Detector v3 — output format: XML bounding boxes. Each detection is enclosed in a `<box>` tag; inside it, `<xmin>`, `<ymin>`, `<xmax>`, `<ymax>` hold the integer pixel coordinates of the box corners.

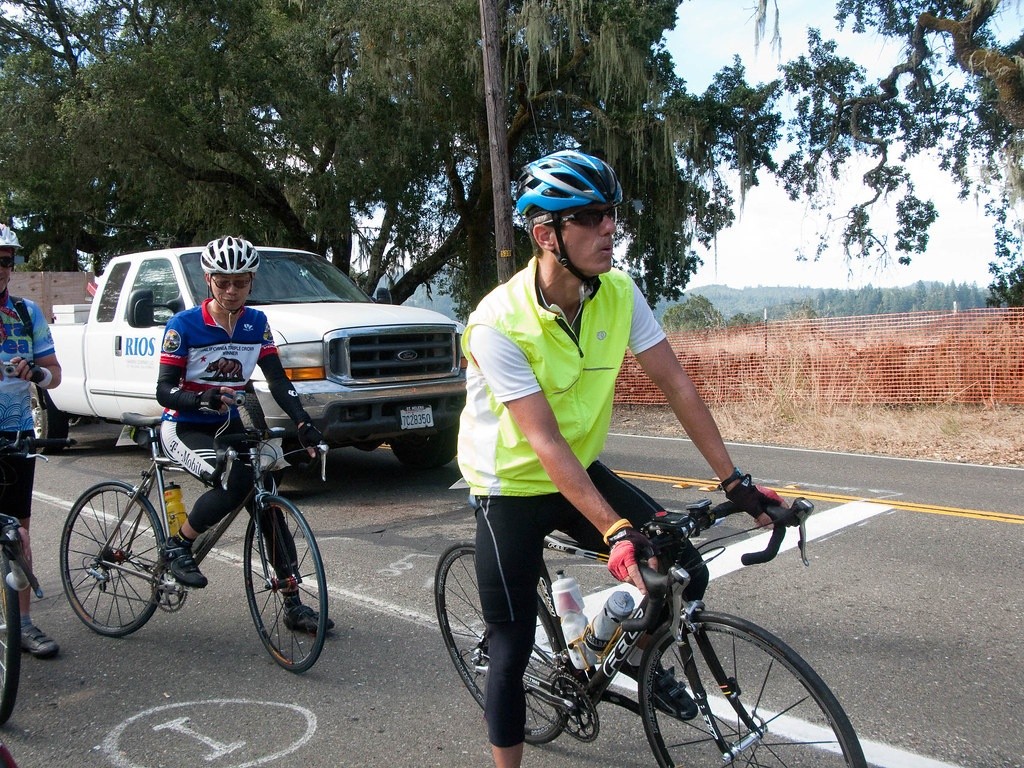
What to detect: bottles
<box><xmin>588</xmin><ymin>592</ymin><xmax>634</xmax><ymax>653</ymax></box>
<box><xmin>163</xmin><ymin>481</ymin><xmax>186</xmax><ymax>537</ymax></box>
<box><xmin>551</xmin><ymin>568</ymin><xmax>598</xmax><ymax>668</ymax></box>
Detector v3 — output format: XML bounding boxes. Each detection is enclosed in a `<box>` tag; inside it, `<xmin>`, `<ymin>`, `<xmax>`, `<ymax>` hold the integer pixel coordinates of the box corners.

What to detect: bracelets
<box><xmin>604</xmin><ymin>516</ymin><xmax>633</xmax><ymax>545</ymax></box>
<box><xmin>33</xmin><ymin>367</ymin><xmax>53</xmax><ymax>388</ymax></box>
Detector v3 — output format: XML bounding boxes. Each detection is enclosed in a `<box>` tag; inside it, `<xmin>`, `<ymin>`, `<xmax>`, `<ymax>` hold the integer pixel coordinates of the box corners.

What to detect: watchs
<box><xmin>717</xmin><ymin>466</ymin><xmax>746</xmax><ymax>492</ymax></box>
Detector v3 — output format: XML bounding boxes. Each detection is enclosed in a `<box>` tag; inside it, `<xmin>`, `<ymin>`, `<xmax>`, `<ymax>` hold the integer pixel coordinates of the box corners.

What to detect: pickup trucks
<box><xmin>46</xmin><ymin>246</ymin><xmax>467</xmax><ymax>490</ymax></box>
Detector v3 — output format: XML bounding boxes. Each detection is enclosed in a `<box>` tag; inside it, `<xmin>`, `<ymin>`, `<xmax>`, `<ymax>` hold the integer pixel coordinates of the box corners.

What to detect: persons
<box><xmin>156</xmin><ymin>235</ymin><xmax>336</xmax><ymax>632</ymax></box>
<box><xmin>0</xmin><ymin>218</ymin><xmax>62</xmax><ymax>659</ymax></box>
<box><xmin>457</xmin><ymin>148</ymin><xmax>795</xmax><ymax>768</ymax></box>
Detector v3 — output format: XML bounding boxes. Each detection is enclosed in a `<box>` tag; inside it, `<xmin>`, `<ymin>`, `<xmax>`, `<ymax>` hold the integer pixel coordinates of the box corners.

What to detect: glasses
<box><xmin>540</xmin><ymin>206</ymin><xmax>615</xmax><ymax>226</ymax></box>
<box><xmin>211</xmin><ymin>276</ymin><xmax>253</xmax><ymax>289</ymax></box>
<box><xmin>0</xmin><ymin>256</ymin><xmax>15</xmax><ymax>267</ymax></box>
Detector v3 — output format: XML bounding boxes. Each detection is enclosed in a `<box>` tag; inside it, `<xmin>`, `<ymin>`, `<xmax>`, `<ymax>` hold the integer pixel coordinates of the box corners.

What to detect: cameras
<box><xmin>0</xmin><ymin>362</ymin><xmax>19</xmax><ymax>378</ymax></box>
<box><xmin>224</xmin><ymin>390</ymin><xmax>247</xmax><ymax>406</ymax></box>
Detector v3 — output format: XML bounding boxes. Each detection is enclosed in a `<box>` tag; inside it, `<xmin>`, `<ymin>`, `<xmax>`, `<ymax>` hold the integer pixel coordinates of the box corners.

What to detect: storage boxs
<box><xmin>52</xmin><ymin>304</ymin><xmax>92</xmax><ymax>323</ymax></box>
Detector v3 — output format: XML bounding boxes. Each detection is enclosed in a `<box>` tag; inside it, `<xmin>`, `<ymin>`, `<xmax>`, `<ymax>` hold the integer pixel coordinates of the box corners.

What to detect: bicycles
<box><xmin>0</xmin><ymin>428</ymin><xmax>75</xmax><ymax>727</ymax></box>
<box><xmin>434</xmin><ymin>496</ymin><xmax>869</xmax><ymax>768</ymax></box>
<box><xmin>59</xmin><ymin>412</ymin><xmax>329</xmax><ymax>674</ymax></box>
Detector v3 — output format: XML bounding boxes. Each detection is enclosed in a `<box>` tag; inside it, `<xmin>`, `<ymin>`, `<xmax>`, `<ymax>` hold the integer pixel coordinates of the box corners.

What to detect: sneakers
<box><xmin>619</xmin><ymin>659</ymin><xmax>698</xmax><ymax>718</ymax></box>
<box><xmin>159</xmin><ymin>537</ymin><xmax>207</xmax><ymax>586</ymax></box>
<box><xmin>283</xmin><ymin>606</ymin><xmax>335</xmax><ymax>631</ymax></box>
<box><xmin>20</xmin><ymin>625</ymin><xmax>57</xmax><ymax>654</ymax></box>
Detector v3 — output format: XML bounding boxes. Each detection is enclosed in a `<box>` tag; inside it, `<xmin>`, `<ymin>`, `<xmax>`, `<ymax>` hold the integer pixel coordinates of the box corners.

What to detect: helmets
<box><xmin>200</xmin><ymin>236</ymin><xmax>261</xmax><ymax>274</ymax></box>
<box><xmin>515</xmin><ymin>151</ymin><xmax>624</xmax><ymax>216</ymax></box>
<box><xmin>0</xmin><ymin>223</ymin><xmax>24</xmax><ymax>250</ymax></box>
